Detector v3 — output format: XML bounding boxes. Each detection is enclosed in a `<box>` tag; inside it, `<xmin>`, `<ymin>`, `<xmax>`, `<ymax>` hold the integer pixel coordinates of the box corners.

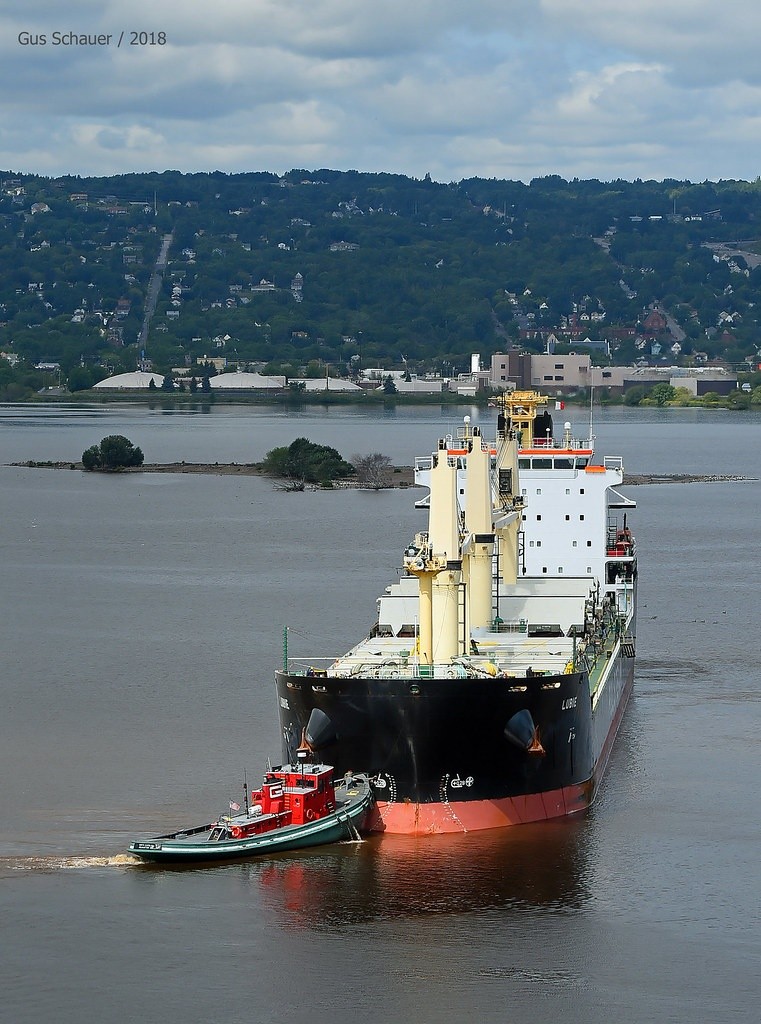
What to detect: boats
<box><xmin>616</xmin><ymin>541</ymin><xmax>630</xmax><ymax>549</ymax></box>
<box><xmin>608</xmin><ymin>550</ymin><xmax>625</xmax><ymax>555</ymax></box>
<box><xmin>126</xmin><ymin>748</ymin><xmax>374</xmax><ymax>865</ymax></box>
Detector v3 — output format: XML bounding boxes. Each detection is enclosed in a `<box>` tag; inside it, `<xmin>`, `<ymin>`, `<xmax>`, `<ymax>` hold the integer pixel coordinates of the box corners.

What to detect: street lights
<box><xmin>358</xmin><ymin>330</ymin><xmax>362</xmax><ymax>378</ymax></box>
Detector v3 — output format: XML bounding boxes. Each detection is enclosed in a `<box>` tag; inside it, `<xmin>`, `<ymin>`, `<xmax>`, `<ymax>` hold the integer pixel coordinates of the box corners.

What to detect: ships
<box><xmin>273</xmin><ymin>385</ymin><xmax>638</xmax><ymax>834</ymax></box>
